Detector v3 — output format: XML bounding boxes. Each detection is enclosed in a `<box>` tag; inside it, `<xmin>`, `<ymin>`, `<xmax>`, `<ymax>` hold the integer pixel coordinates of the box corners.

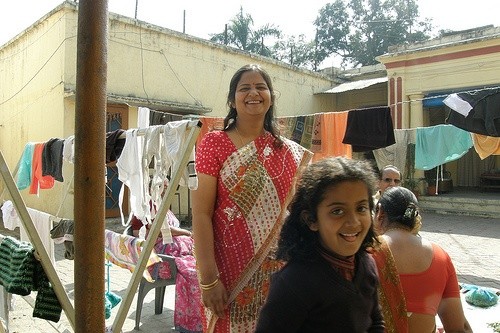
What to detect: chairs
<box><xmin>122</xmin><ymin>226</ymin><xmax>176</xmax><ymax>331</ymax></box>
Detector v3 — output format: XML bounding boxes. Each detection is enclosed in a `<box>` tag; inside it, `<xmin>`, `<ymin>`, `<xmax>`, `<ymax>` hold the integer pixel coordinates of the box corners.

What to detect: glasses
<box><xmin>381</xmin><ymin>178</ymin><xmax>401</xmax><ymax>183</ymax></box>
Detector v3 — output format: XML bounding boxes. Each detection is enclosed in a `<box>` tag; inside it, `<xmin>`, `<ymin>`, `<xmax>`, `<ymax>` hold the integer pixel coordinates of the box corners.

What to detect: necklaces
<box><xmin>233</xmin><ymin>123</ymin><xmax>250</xmax><ymax>147</ymax></box>
<box><xmin>389</xmin><ymin>225</ymin><xmax>411</xmax><ymax>232</ymax></box>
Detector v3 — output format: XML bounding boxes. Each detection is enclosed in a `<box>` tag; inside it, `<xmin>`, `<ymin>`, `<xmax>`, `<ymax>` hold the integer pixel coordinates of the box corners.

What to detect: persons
<box><xmin>371</xmin><ymin>165</ymin><xmax>424</xmax><ymax>236</ymax></box>
<box><xmin>366</xmin><ymin>186</ymin><xmax>474</xmax><ymax>333</ymax></box>
<box><xmin>189</xmin><ymin>64</ymin><xmax>314</xmax><ymax>333</ymax></box>
<box><xmin>254</xmin><ymin>154</ymin><xmax>384</xmax><ymax>333</ymax></box>
<box><xmin>133</xmin><ymin>174</ymin><xmax>203</xmax><ymax>333</ymax></box>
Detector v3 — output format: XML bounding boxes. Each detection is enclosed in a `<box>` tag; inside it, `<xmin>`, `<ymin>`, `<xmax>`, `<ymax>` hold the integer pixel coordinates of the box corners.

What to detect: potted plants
<box><xmin>430</xmin><ymin>176</ymin><xmax>436</xmax><ymax>195</ymax></box>
<box><xmin>404</xmin><ymin>179</ymin><xmax>419</xmax><ymax>200</ymax></box>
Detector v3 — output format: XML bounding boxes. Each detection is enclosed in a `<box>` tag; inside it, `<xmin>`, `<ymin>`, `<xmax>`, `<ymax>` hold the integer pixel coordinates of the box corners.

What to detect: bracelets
<box><xmin>198</xmin><ymin>278</ymin><xmax>220</xmax><ymax>291</ymax></box>
<box><xmin>190</xmin><ymin>231</ymin><xmax>193</xmax><ymax>238</ymax></box>
